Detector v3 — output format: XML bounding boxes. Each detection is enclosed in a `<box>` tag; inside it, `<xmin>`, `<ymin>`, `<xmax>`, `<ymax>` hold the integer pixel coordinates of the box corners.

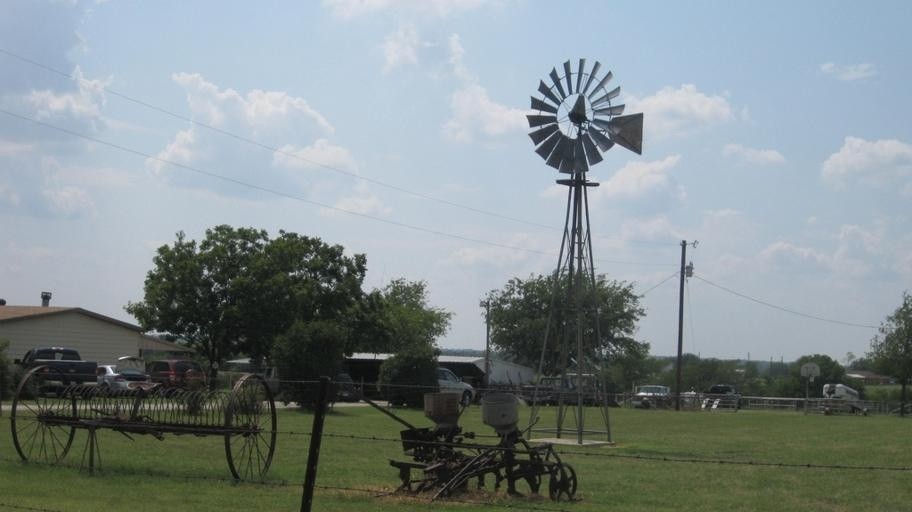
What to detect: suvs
<box><xmin>634</xmin><ymin>385</ymin><xmax>671</xmax><ymax>406</ymax></box>
<box><xmin>147</xmin><ymin>359</ymin><xmax>206</xmax><ymax>388</ymax></box>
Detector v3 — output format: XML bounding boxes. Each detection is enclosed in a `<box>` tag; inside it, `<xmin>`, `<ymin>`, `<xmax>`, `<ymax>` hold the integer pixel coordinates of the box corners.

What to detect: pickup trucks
<box><xmin>14</xmin><ymin>343</ymin><xmax>97</xmax><ymax>399</ymax></box>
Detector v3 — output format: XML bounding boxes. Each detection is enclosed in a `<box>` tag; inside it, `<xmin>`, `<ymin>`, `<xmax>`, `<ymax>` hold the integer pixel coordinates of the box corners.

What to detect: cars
<box><xmin>703</xmin><ymin>384</ymin><xmax>741</xmax><ymax>408</ymax></box>
<box><xmin>98</xmin><ymin>355</ymin><xmax>158</xmax><ymax>394</ymax></box>
<box><xmin>436</xmin><ymin>366</ymin><xmax>476</xmax><ymax>406</ymax></box>
